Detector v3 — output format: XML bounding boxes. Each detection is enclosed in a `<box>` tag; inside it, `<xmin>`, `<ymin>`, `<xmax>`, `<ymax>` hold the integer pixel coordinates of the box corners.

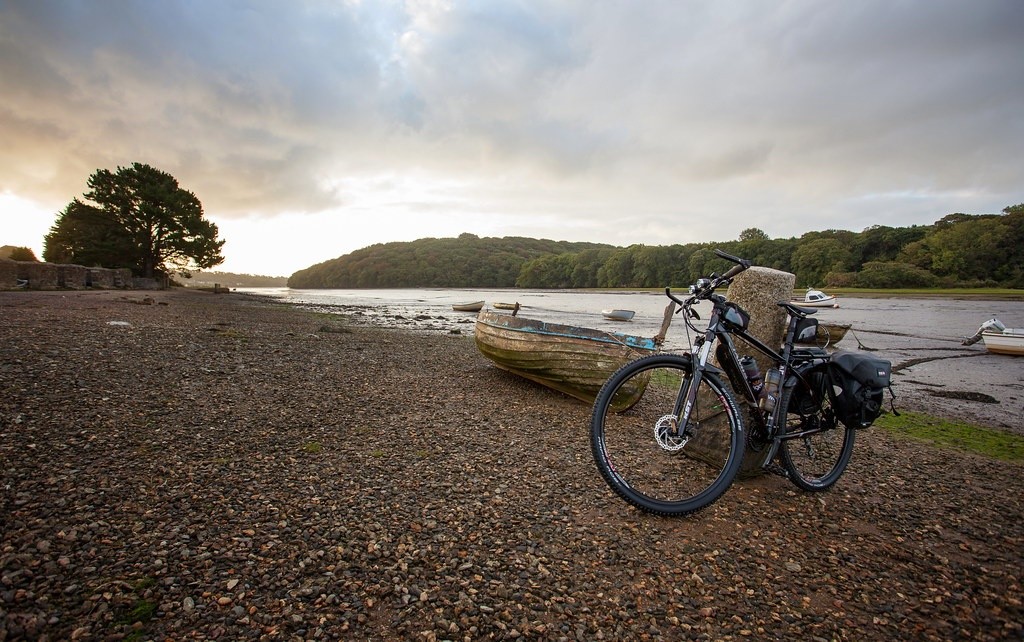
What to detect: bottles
<box><xmin>759</xmin><ymin>362</ymin><xmax>782</xmax><ymax>411</ymax></box>
<box><xmin>739</xmin><ymin>354</ymin><xmax>765</xmax><ymax>398</ymax></box>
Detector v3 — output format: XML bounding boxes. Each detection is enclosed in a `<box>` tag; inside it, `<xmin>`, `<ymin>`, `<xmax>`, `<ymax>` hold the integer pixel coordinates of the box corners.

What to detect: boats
<box><xmin>787</xmin><ymin>285</ymin><xmax>837</xmax><ymax>307</ymax></box>
<box><xmin>601</xmin><ymin>306</ymin><xmax>636</xmax><ymax>322</ymax></box>
<box><xmin>966</xmin><ymin>321</ymin><xmax>1023</xmax><ymax>360</ymax></box>
<box><xmin>493</xmin><ymin>303</ymin><xmax>522</xmax><ymax>310</ymax></box>
<box><xmin>452</xmin><ymin>300</ymin><xmax>485</xmax><ymax>313</ymax></box>
<box><xmin>475</xmin><ymin>298</ymin><xmax>681</xmax><ymax>413</ymax></box>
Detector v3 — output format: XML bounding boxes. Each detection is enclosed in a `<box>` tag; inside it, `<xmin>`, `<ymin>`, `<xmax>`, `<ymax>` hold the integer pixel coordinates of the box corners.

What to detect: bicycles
<box><xmin>588</xmin><ymin>249</ymin><xmax>864</xmax><ymax>518</ymax></box>
